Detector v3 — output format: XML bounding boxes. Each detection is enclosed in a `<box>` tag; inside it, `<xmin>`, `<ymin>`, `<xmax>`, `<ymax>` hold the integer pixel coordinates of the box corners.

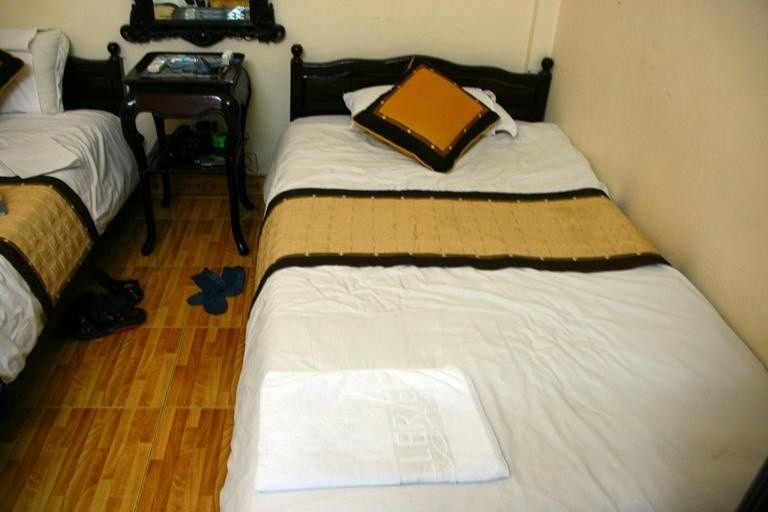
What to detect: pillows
<box><xmin>350</xmin><ymin>55</ymin><xmax>501</xmax><ymax>173</ymax></box>
<box><xmin>1</xmin><ymin>50</ymin><xmax>26</xmax><ymax>92</ymax></box>
<box><xmin>1</xmin><ymin>24</ymin><xmax>72</xmax><ymax>118</ymax></box>
<box><xmin>340</xmin><ymin>84</ymin><xmax>520</xmax><ymax>138</ymax></box>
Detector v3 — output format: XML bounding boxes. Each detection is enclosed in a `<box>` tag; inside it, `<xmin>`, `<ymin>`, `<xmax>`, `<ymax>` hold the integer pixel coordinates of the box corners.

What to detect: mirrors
<box><xmin>117</xmin><ymin>1</ymin><xmax>289</xmax><ymax>45</ymax></box>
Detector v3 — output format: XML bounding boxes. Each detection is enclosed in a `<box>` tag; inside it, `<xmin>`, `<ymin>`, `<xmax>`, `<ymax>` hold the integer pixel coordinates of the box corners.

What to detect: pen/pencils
<box><xmin>200</xmin><ymin>56</ymin><xmax>216</xmax><ymax>76</ymax></box>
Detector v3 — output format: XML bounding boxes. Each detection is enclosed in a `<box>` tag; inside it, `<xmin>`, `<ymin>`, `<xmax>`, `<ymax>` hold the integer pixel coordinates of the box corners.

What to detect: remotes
<box><xmin>147</xmin><ymin>55</ymin><xmax>166</xmax><ymax>74</ymax></box>
<box><xmin>185</xmin><ymin>7</ymin><xmax>196</xmax><ymax>21</ymax></box>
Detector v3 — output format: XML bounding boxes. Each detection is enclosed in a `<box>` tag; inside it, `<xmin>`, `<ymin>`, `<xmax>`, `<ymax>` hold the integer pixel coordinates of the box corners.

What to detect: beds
<box><xmin>218</xmin><ymin>43</ymin><xmax>767</xmax><ymax>512</ymax></box>
<box><xmin>0</xmin><ymin>42</ymin><xmax>140</xmax><ymax>411</ymax></box>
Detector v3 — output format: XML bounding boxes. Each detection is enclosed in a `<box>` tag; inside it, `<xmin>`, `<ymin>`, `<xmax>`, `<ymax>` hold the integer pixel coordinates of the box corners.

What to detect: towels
<box><xmin>256</xmin><ymin>362</ymin><xmax>507</xmax><ymax>492</ymax></box>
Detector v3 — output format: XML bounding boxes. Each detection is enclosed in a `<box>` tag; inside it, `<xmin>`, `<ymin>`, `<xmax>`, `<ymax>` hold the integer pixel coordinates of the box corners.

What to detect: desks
<box><xmin>120</xmin><ymin>51</ymin><xmax>257</xmax><ymax>255</ymax></box>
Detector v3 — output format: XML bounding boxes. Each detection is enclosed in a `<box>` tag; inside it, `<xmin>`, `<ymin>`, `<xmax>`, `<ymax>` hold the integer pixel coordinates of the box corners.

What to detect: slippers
<box><xmin>68</xmin><ymin>306</ymin><xmax>147</xmax><ymax>341</ymax></box>
<box><xmin>88</xmin><ymin>277</ymin><xmax>144</xmax><ymax>310</ymax></box>
